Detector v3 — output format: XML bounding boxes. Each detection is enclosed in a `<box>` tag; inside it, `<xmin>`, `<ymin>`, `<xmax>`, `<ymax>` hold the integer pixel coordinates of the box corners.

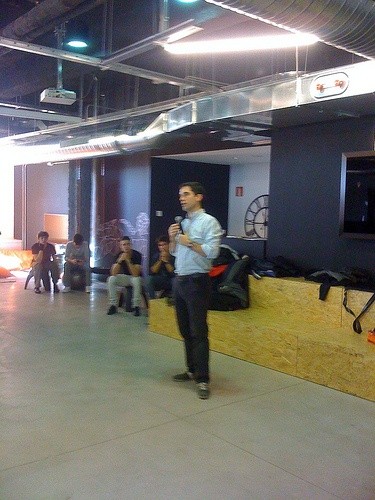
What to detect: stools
<box><xmin>24</xmin><ymin>269</ymin><xmax>51</xmax><ymax>291</ymax></box>
<box><xmin>118</xmin><ymin>287</ymin><xmax>148</xmax><ymax>312</ymax></box>
<box><xmin>62</xmin><ymin>267</ymin><xmax>91</xmax><ymax>289</ymax></box>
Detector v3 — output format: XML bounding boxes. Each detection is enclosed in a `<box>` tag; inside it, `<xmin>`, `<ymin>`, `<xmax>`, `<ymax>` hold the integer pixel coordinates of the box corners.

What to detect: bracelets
<box><xmin>188</xmin><ymin>240</ymin><xmax>193</xmax><ymax>249</ymax></box>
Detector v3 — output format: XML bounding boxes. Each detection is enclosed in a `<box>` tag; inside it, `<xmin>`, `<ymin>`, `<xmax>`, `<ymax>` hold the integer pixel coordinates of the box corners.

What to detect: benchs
<box><xmin>148</xmin><ymin>274</ymin><xmax>375</xmax><ymax>402</ymax></box>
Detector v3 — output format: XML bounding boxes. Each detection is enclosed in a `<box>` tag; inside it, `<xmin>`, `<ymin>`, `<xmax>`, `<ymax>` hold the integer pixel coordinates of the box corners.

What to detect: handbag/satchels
<box><xmin>343</xmin><ymin>268</ymin><xmax>375</xmax><ymax>293</ymax></box>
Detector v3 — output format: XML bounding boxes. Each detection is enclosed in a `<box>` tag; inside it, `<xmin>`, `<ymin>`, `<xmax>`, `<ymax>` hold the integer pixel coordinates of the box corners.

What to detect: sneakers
<box><xmin>107</xmin><ymin>306</ymin><xmax>116</xmax><ymax>315</ymax></box>
<box><xmin>197</xmin><ymin>382</ymin><xmax>209</xmax><ymax>399</ymax></box>
<box><xmin>62</xmin><ymin>286</ymin><xmax>71</xmax><ymax>293</ymax></box>
<box><xmin>54</xmin><ymin>286</ymin><xmax>59</xmax><ymax>293</ymax></box>
<box><xmin>172</xmin><ymin>372</ymin><xmax>193</xmax><ymax>381</ymax></box>
<box><xmin>85</xmin><ymin>286</ymin><xmax>91</xmax><ymax>293</ymax></box>
<box><xmin>133</xmin><ymin>306</ymin><xmax>140</xmax><ymax>316</ymax></box>
<box><xmin>34</xmin><ymin>288</ymin><xmax>41</xmax><ymax>293</ymax></box>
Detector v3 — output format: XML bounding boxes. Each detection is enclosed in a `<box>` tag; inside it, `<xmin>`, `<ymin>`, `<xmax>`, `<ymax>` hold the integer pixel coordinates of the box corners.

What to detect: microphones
<box><xmin>175</xmin><ymin>216</ymin><xmax>184</xmax><ymax>235</ymax></box>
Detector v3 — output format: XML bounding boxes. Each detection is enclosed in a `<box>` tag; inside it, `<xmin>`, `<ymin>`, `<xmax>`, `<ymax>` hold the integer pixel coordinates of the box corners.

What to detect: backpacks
<box><xmin>208</xmin><ymin>260</ymin><xmax>249</xmax><ymax>311</ymax></box>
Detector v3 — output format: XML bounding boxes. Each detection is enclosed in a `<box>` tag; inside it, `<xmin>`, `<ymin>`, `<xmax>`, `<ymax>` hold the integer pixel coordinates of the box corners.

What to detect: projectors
<box><xmin>40</xmin><ymin>88</ymin><xmax>76</xmax><ymax>105</ymax></box>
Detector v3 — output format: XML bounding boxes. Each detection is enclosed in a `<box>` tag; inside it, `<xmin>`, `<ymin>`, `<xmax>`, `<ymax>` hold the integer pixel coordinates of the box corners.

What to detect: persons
<box><xmin>147</xmin><ymin>234</ymin><xmax>175</xmax><ymax>299</ymax></box>
<box><xmin>32</xmin><ymin>231</ymin><xmax>60</xmax><ymax>293</ymax></box>
<box><xmin>62</xmin><ymin>234</ymin><xmax>91</xmax><ymax>293</ymax></box>
<box><xmin>168</xmin><ymin>181</ymin><xmax>220</xmax><ymax>400</ymax></box>
<box><xmin>106</xmin><ymin>236</ymin><xmax>142</xmax><ymax>316</ymax></box>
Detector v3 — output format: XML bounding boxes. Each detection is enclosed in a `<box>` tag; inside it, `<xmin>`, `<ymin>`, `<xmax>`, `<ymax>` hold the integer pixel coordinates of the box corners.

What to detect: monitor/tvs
<box><xmin>339</xmin><ymin>151</ymin><xmax>375</xmax><ymax>239</ymax></box>
